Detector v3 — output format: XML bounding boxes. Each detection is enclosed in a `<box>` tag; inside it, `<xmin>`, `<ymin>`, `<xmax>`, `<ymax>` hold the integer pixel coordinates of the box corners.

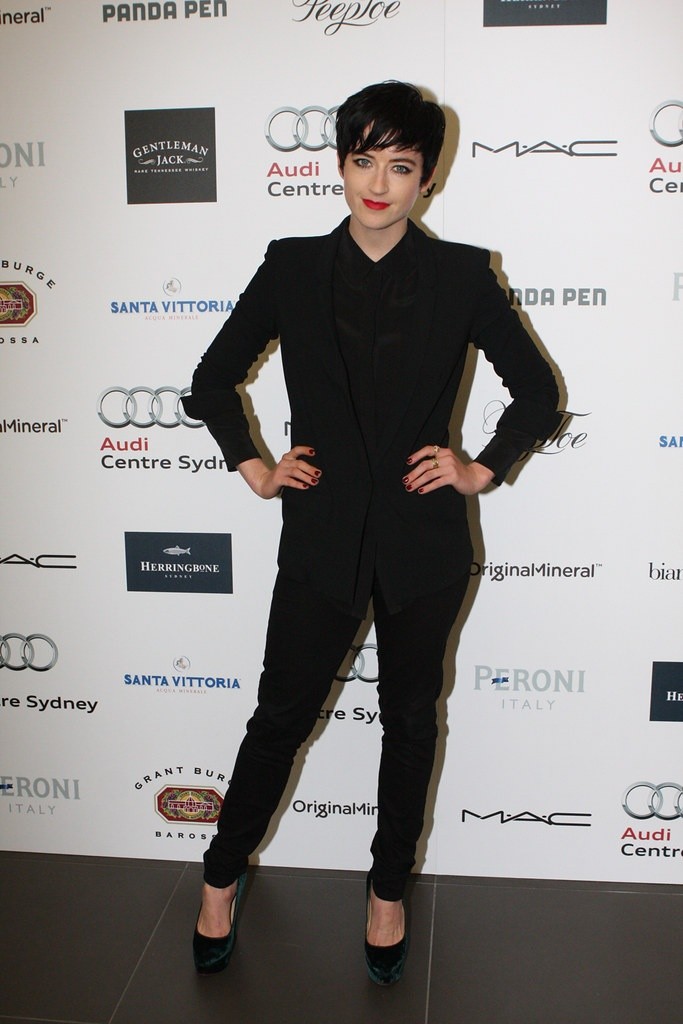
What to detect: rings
<box><xmin>433</xmin><ymin>445</ymin><xmax>440</xmax><ymax>456</ymax></box>
<box><xmin>433</xmin><ymin>458</ymin><xmax>439</xmax><ymax>469</ymax></box>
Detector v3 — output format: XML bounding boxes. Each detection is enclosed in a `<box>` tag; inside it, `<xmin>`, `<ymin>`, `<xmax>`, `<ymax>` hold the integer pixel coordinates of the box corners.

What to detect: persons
<box><xmin>193</xmin><ymin>82</ymin><xmax>560</xmax><ymax>987</ymax></box>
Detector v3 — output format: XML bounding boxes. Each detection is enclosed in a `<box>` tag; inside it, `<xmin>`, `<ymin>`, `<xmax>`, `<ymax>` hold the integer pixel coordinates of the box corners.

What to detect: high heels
<box><xmin>363</xmin><ymin>868</ymin><xmax>409</xmax><ymax>987</ymax></box>
<box><xmin>193</xmin><ymin>869</ymin><xmax>247</xmax><ymax>977</ymax></box>
